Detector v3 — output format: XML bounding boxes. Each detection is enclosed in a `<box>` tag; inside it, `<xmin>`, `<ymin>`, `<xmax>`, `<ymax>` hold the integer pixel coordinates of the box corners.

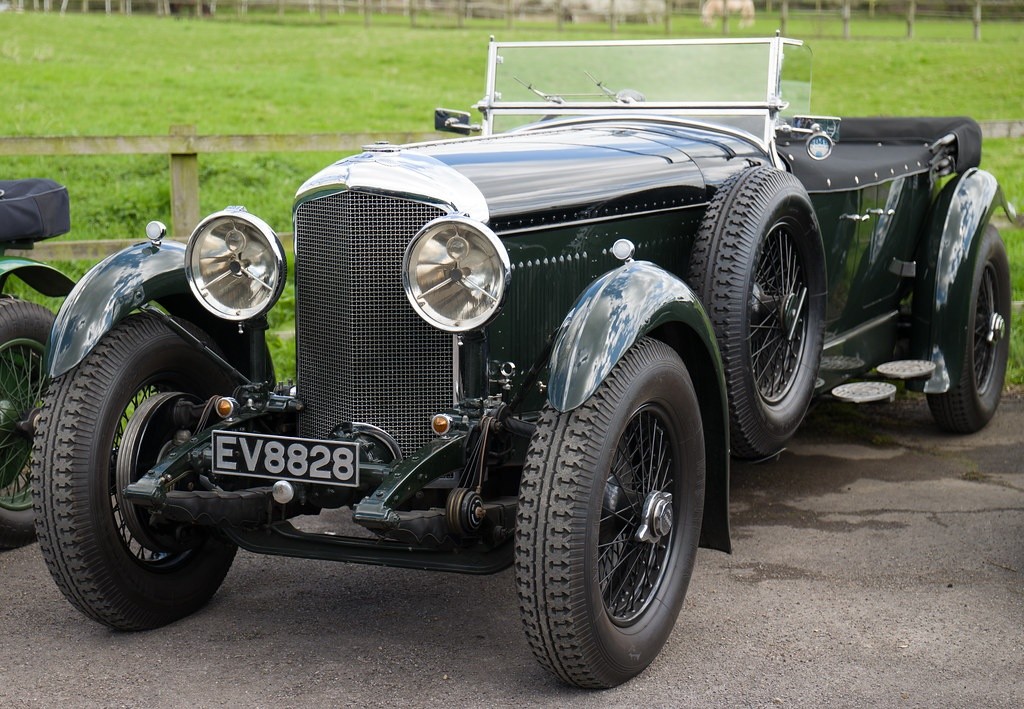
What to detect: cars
<box><xmin>0</xmin><ymin>178</ymin><xmax>77</xmax><ymax>549</ymax></box>
<box><xmin>32</xmin><ymin>29</ymin><xmax>1016</xmax><ymax>689</ymax></box>
